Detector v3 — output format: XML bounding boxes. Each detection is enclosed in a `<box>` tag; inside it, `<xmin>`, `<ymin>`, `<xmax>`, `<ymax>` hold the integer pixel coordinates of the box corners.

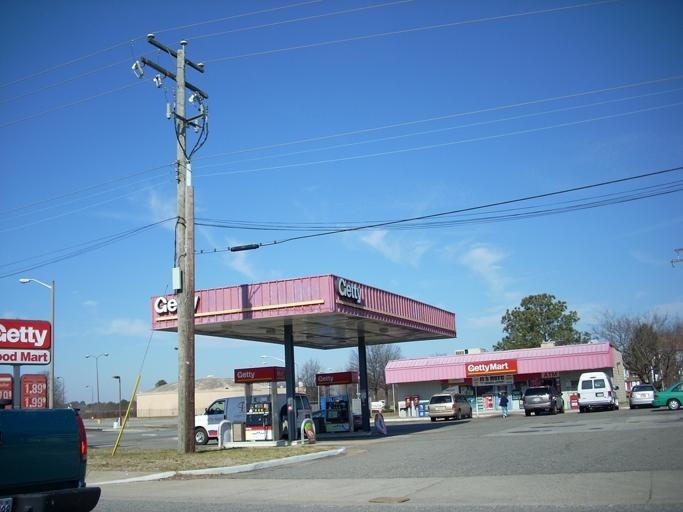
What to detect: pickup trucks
<box><xmin>0</xmin><ymin>407</ymin><xmax>101</xmax><ymax>512</ymax></box>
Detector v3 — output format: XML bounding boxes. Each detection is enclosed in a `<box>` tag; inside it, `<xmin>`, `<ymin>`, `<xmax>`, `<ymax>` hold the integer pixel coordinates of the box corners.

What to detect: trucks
<box><xmin>194</xmin><ymin>392</ymin><xmax>312</xmax><ymax>445</ymax></box>
<box><xmin>576</xmin><ymin>371</ymin><xmax>619</xmax><ymax>412</ymax></box>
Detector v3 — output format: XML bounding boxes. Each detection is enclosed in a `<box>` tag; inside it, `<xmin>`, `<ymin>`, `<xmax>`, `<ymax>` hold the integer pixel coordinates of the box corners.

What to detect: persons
<box><xmin>498</xmin><ymin>392</ymin><xmax>508</xmax><ymax>420</ymax></box>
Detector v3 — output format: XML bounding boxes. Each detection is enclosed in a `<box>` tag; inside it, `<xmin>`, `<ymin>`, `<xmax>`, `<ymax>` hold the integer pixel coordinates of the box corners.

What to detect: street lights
<box><xmin>85</xmin><ymin>384</ymin><xmax>93</xmax><ymax>410</ymax></box>
<box><xmin>17</xmin><ymin>277</ymin><xmax>55</xmax><ymax>410</ymax></box>
<box><xmin>85</xmin><ymin>352</ymin><xmax>108</xmax><ymax>410</ymax></box>
<box><xmin>56</xmin><ymin>376</ymin><xmax>63</xmax><ymax>408</ymax></box>
<box><xmin>259</xmin><ymin>354</ymin><xmax>285</xmax><ymax>365</ymax></box>
<box><xmin>112</xmin><ymin>375</ymin><xmax>122</xmax><ymax>428</ymax></box>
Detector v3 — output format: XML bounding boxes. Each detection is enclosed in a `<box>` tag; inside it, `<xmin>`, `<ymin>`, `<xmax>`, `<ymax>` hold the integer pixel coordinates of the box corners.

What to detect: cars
<box><xmin>652</xmin><ymin>381</ymin><xmax>683</xmax><ymax>410</ymax></box>
<box><xmin>312</xmin><ymin>409</ymin><xmax>361</xmax><ymax>433</ymax></box>
<box><xmin>627</xmin><ymin>383</ymin><xmax>660</xmax><ymax>409</ymax></box>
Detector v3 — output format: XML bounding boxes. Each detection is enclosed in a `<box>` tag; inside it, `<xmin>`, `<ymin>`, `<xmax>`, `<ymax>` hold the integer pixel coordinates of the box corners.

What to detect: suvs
<box><xmin>522</xmin><ymin>385</ymin><xmax>564</xmax><ymax>416</ymax></box>
<box><xmin>428</xmin><ymin>393</ymin><xmax>473</xmax><ymax>421</ymax></box>
